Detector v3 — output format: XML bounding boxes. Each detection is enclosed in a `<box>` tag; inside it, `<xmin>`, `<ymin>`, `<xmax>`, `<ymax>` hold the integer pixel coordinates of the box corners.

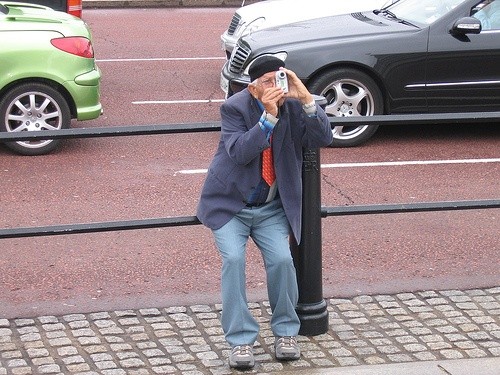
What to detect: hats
<box><xmin>248</xmin><ymin>56</ymin><xmax>285</xmax><ymax>83</ymax></box>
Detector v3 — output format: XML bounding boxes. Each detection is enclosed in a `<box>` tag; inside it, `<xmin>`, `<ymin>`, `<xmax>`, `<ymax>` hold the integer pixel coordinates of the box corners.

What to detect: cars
<box><xmin>0</xmin><ymin>1</ymin><xmax>105</xmax><ymax>156</ymax></box>
<box><xmin>220</xmin><ymin>1</ymin><xmax>391</xmax><ymax>63</ymax></box>
<box><xmin>218</xmin><ymin>0</ymin><xmax>500</xmax><ymax>149</ymax></box>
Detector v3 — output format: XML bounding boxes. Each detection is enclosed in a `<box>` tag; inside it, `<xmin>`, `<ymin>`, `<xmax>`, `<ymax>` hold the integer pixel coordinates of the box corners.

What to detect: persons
<box><xmin>195</xmin><ymin>56</ymin><xmax>334</xmax><ymax>370</ymax></box>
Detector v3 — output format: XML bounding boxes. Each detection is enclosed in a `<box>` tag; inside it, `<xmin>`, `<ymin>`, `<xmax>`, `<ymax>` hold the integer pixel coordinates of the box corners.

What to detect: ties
<box><xmin>262</xmin><ymin>134</ymin><xmax>276</xmax><ymax>187</ymax></box>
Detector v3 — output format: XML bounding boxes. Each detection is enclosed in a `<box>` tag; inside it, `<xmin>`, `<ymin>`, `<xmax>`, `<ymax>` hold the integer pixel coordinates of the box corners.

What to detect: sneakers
<box><xmin>229</xmin><ymin>346</ymin><xmax>254</xmax><ymax>368</ymax></box>
<box><xmin>274</xmin><ymin>336</ymin><xmax>301</xmax><ymax>359</ymax></box>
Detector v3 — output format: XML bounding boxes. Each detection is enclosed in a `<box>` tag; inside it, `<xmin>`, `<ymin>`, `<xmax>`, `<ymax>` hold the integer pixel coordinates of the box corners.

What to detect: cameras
<box><xmin>274</xmin><ymin>71</ymin><xmax>289</xmax><ymax>93</ymax></box>
